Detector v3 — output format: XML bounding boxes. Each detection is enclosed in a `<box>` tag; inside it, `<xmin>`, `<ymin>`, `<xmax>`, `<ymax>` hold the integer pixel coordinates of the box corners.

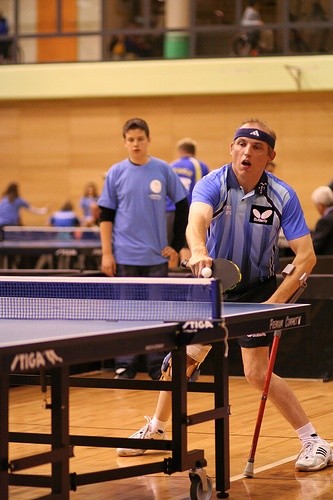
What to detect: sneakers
<box><xmin>115</xmin><ymin>416</ymin><xmax>166</xmax><ymax>456</ymax></box>
<box><xmin>294</xmin><ymin>440</ymin><xmax>332</xmax><ymax>470</ymax></box>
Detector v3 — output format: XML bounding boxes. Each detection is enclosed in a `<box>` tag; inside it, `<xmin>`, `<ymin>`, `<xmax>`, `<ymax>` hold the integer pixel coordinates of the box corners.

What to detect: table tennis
<box><xmin>202</xmin><ymin>267</ymin><xmax>212</xmax><ymax>277</ymax></box>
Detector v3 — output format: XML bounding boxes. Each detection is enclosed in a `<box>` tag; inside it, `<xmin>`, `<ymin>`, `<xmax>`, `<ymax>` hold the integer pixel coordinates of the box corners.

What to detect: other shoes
<box><xmin>147</xmin><ymin>366</ymin><xmax>162</xmax><ymax>380</ymax></box>
<box><xmin>112</xmin><ymin>366</ymin><xmax>137</xmax><ymax>380</ymax></box>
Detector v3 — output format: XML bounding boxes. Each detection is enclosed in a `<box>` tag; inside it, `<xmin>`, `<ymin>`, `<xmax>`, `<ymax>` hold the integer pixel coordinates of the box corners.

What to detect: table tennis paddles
<box><xmin>180</xmin><ymin>258</ymin><xmax>240</xmax><ymax>289</ymax></box>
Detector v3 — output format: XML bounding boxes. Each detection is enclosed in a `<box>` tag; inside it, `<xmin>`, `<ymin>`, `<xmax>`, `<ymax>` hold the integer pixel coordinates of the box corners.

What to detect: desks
<box><xmin>0</xmin><ymin>275</ymin><xmax>313</xmax><ymax>500</ymax></box>
<box><xmin>0</xmin><ymin>225</ymin><xmax>102</xmax><ymax>267</ymax></box>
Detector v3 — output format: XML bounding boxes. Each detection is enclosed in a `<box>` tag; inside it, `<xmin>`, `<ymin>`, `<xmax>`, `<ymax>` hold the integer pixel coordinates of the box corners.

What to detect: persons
<box><xmin>80</xmin><ymin>181</ymin><xmax>99</xmax><ymax>231</ymax></box>
<box><xmin>115</xmin><ymin>119</ymin><xmax>333</xmax><ymax>473</ymax></box>
<box><xmin>282</xmin><ymin>185</ymin><xmax>333</xmax><ymax>256</ymax></box>
<box><xmin>51</xmin><ymin>197</ymin><xmax>80</xmax><ymax>230</ymax></box>
<box><xmin>263</xmin><ymin>160</ymin><xmax>280</xmax><ymax>178</ymax></box>
<box><xmin>165</xmin><ymin>136</ymin><xmax>210</xmax><ymax>190</ymax></box>
<box><xmin>0</xmin><ymin>0</ymin><xmax>274</xmax><ymax>58</ymax></box>
<box><xmin>1</xmin><ymin>181</ymin><xmax>50</xmax><ymax>229</ymax></box>
<box><xmin>96</xmin><ymin>119</ymin><xmax>192</xmax><ymax>381</ymax></box>
